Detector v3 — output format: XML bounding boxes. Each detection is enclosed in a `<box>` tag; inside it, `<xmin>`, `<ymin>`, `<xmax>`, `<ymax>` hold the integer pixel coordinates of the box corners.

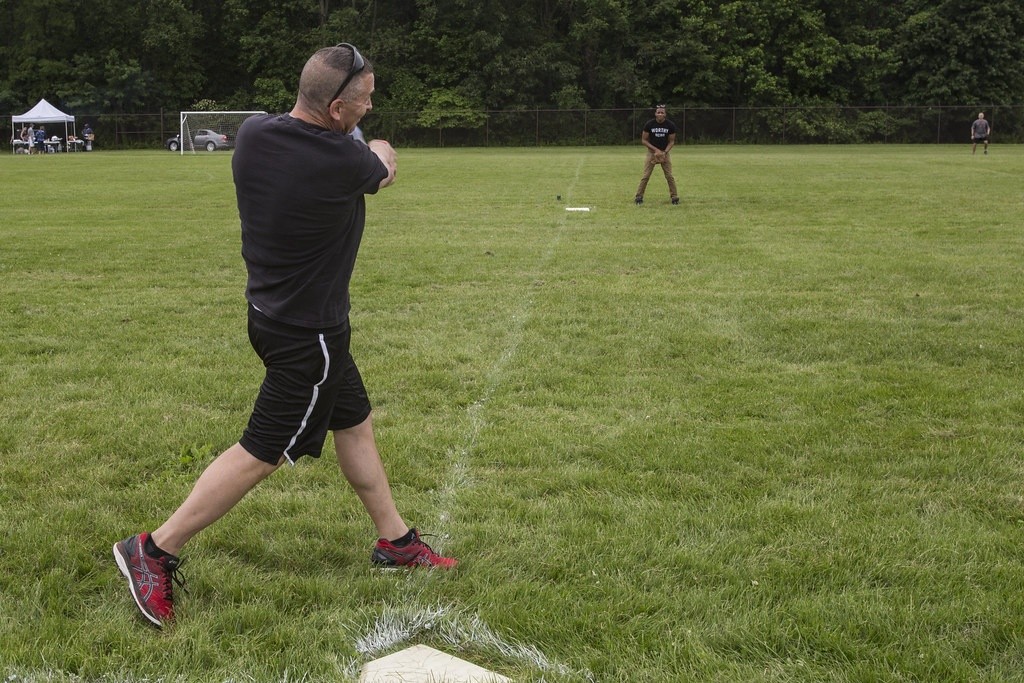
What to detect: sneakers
<box><xmin>373</xmin><ymin>528</ymin><xmax>458</xmax><ymax>572</ymax></box>
<box><xmin>113</xmin><ymin>533</ymin><xmax>189</xmax><ymax>627</ymax></box>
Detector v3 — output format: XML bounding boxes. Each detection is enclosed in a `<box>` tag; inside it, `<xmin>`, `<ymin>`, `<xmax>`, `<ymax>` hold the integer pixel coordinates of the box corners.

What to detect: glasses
<box><xmin>327</xmin><ymin>43</ymin><xmax>365</xmax><ymax>108</ymax></box>
<box><xmin>656</xmin><ymin>104</ymin><xmax>667</xmax><ymax>109</ymax></box>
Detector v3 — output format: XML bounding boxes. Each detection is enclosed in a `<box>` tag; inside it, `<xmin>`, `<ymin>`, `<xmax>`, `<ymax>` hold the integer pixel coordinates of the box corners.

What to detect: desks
<box><xmin>66</xmin><ymin>140</ymin><xmax>85</xmax><ymax>152</ymax></box>
<box><xmin>12</xmin><ymin>140</ymin><xmax>60</xmax><ymax>154</ymax></box>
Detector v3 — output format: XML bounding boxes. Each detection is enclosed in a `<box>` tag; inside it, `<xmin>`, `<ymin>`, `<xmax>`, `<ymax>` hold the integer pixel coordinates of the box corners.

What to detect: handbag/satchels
<box><xmin>29</xmin><ymin>136</ymin><xmax>36</xmax><ymax>147</ymax></box>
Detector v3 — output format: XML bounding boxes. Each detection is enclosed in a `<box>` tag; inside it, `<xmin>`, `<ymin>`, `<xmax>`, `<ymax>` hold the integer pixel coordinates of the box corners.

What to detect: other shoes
<box><xmin>635</xmin><ymin>198</ymin><xmax>643</xmax><ymax>205</ymax></box>
<box><xmin>672</xmin><ymin>198</ymin><xmax>679</xmax><ymax>205</ymax></box>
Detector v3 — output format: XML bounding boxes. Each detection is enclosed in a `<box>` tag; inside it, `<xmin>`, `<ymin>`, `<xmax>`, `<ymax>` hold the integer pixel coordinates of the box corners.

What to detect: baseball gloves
<box><xmin>651</xmin><ymin>151</ymin><xmax>667</xmax><ymax>165</ymax></box>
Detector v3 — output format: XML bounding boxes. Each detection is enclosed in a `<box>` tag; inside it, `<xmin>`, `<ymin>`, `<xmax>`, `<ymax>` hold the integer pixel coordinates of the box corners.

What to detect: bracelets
<box><xmin>664</xmin><ymin>151</ymin><xmax>667</xmax><ymax>154</ymax></box>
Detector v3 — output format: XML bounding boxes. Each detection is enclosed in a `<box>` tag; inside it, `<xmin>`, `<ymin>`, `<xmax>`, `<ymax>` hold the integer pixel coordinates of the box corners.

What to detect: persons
<box><xmin>35</xmin><ymin>126</ymin><xmax>45</xmax><ymax>154</ymax></box>
<box><xmin>635</xmin><ymin>105</ymin><xmax>680</xmax><ymax>205</ymax></box>
<box><xmin>9</xmin><ymin>127</ymin><xmax>21</xmax><ymax>144</ymax></box>
<box><xmin>81</xmin><ymin>124</ymin><xmax>93</xmax><ymax>152</ymax></box>
<box><xmin>112</xmin><ymin>42</ymin><xmax>459</xmax><ymax>630</ymax></box>
<box><xmin>27</xmin><ymin>123</ymin><xmax>35</xmax><ymax>154</ymax></box>
<box><xmin>51</xmin><ymin>132</ymin><xmax>62</xmax><ymax>152</ymax></box>
<box><xmin>68</xmin><ymin>132</ymin><xmax>75</xmax><ymax>151</ymax></box>
<box><xmin>21</xmin><ymin>126</ymin><xmax>27</xmax><ymax>149</ymax></box>
<box><xmin>971</xmin><ymin>113</ymin><xmax>990</xmax><ymax>155</ymax></box>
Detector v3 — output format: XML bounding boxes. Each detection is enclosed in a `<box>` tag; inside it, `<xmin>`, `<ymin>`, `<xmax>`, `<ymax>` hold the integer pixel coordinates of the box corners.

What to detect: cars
<box><xmin>9</xmin><ymin>129</ymin><xmax>48</xmax><ymax>152</ymax></box>
<box><xmin>165</xmin><ymin>128</ymin><xmax>230</xmax><ymax>152</ymax></box>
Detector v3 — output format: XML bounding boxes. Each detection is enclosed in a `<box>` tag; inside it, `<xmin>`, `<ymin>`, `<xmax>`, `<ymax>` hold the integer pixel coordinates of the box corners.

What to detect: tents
<box><xmin>12</xmin><ymin>98</ymin><xmax>76</xmax><ymax>154</ymax></box>
